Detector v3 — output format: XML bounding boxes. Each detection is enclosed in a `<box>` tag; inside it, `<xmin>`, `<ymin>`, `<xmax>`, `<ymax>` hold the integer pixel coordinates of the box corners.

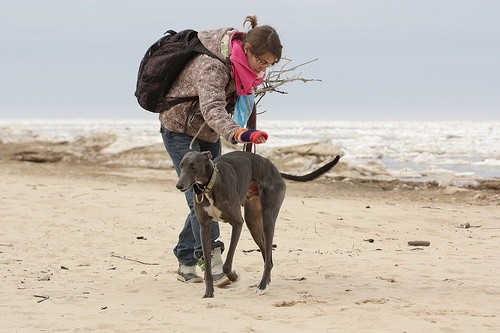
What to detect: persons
<box><xmin>158</xmin><ymin>16</ymin><xmax>283</xmax><ymax>288</ymax></box>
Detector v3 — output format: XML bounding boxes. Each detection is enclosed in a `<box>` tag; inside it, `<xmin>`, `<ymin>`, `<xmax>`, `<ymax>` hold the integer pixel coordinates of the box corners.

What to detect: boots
<box><xmin>176</xmin><ymin>262</ymin><xmax>204</xmax><ymax>283</ymax></box>
<box><xmin>196</xmin><ymin>247</ymin><xmax>230</xmax><ymax>286</ymax></box>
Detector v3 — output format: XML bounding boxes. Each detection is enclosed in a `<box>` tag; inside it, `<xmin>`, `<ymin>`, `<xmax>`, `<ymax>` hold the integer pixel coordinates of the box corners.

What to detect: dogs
<box><xmin>176</xmin><ymin>150</ymin><xmax>341</xmax><ymax>298</ymax></box>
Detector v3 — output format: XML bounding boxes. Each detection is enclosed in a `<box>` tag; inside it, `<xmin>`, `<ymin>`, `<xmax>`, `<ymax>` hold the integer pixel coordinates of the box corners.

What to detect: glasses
<box><xmin>249</xmin><ymin>48</ymin><xmax>274</xmax><ymax>68</ymax></box>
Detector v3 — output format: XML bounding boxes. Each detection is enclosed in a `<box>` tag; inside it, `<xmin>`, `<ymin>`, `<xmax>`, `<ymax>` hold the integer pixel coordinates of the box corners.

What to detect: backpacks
<box><xmin>134</xmin><ymin>29</ymin><xmax>232</xmax><ymax>113</ymax></box>
